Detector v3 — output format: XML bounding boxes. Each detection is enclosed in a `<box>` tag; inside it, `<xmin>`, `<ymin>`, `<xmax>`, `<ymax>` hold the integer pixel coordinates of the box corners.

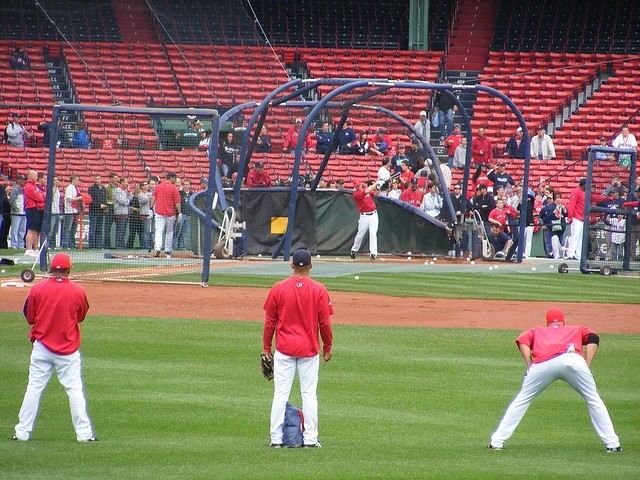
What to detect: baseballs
<box><xmin>407</xmin><ymin>257</ymin><xmax>411</xmax><ymax>260</ymax></box>
<box><xmin>166</xmin><ymin>255</ymin><xmax>170</xmax><ymax>258</ymax></box>
<box><xmin>429</xmin><ymin>261</ymin><xmax>434</xmax><ymax>264</ymax></box>
<box><xmin>489</xmin><ymin>266</ymin><xmax>493</xmax><ymax>270</ymax></box>
<box><xmin>550</xmin><ymin>265</ymin><xmax>554</xmax><ymax>268</ymax></box>
<box><xmin>532</xmin><ymin>267</ymin><xmax>536</xmax><ymax>271</ymax></box>
<box><xmin>466</xmin><ymin>258</ymin><xmax>470</xmax><ymax>261</ymax></box>
<box><xmin>354</xmin><ymin>276</ymin><xmax>359</xmax><ymax>280</ymax></box>
<box><xmin>424</xmin><ymin>261</ymin><xmax>428</xmax><ymax>265</ymax></box>
<box><xmin>0</xmin><ymin>269</ymin><xmax>6</xmax><ymax>273</ymax></box>
<box><xmin>494</xmin><ymin>264</ymin><xmax>498</xmax><ymax>269</ymax></box>
<box><xmin>433</xmin><ymin>257</ymin><xmax>437</xmax><ymax>261</ymax></box>
<box><xmin>258</xmin><ymin>253</ymin><xmax>262</xmax><ymax>257</ymax></box>
<box><xmin>317</xmin><ymin>254</ymin><xmax>320</xmax><ymax>258</ymax></box>
<box><xmin>471</xmin><ymin>261</ymin><xmax>475</xmax><ymax>264</ymax></box>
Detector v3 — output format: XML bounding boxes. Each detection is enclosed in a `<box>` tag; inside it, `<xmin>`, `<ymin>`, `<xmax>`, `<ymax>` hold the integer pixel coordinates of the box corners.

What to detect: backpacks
<box><xmin>4</xmin><ymin>123</ymin><xmax>23</xmax><ymax>139</ymax></box>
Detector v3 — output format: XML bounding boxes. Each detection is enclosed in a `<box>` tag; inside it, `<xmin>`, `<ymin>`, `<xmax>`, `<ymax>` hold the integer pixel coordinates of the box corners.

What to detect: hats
<box><xmin>375</xmin><ymin>128</ymin><xmax>384</xmax><ymax>134</ymax></box>
<box><xmin>411</xmin><ymin>177</ymin><xmax>418</xmax><ymax>184</ymax></box>
<box><xmin>422</xmin><ymin>170</ymin><xmax>427</xmax><ymax>175</ymax></box>
<box><xmin>353</xmin><ymin>178</ymin><xmax>362</xmax><ymax>187</ymax></box>
<box><xmin>13</xmin><ymin>114</ymin><xmax>22</xmax><ymax>118</ymax></box>
<box><xmin>174</xmin><ymin>129</ymin><xmax>183</xmax><ymax>134</ymax></box>
<box><xmin>17</xmin><ymin>175</ymin><xmax>27</xmax><ymax>180</ymax></box>
<box><xmin>167</xmin><ymin>172</ymin><xmax>176</xmax><ymax>176</ymax></box>
<box><xmin>198</xmin><ymin>129</ymin><xmax>205</xmax><ymax>133</ymax></box>
<box><xmin>454</xmin><ymin>124</ymin><xmax>461</xmax><ymax>128</ymax></box>
<box><xmin>194</xmin><ymin>120</ymin><xmax>204</xmax><ymax>126</ymax></box>
<box><xmin>420</xmin><ymin>110</ymin><xmax>426</xmax><ymax>117</ymax></box>
<box><xmin>535</xmin><ymin>125</ymin><xmax>544</xmax><ymax>129</ymax></box>
<box><xmin>337</xmin><ymin>179</ymin><xmax>344</xmax><ymax>184</ymax></box>
<box><xmin>51</xmin><ymin>254</ymin><xmax>73</xmax><ymax>269</ymax></box>
<box><xmin>499</xmin><ymin>162</ymin><xmax>506</xmax><ymax>166</ymax></box>
<box><xmin>480</xmin><ymin>162</ymin><xmax>486</xmax><ymax>167</ymax></box>
<box><xmin>600</xmin><ymin>137</ymin><xmax>606</xmax><ymax>141</ymax></box>
<box><xmin>517</xmin><ymin>127</ymin><xmax>522</xmax><ymax>132</ymax></box>
<box><xmin>442</xmin><ymin>75</ymin><xmax>449</xmax><ymax>82</ymax></box>
<box><xmin>399</xmin><ymin>145</ymin><xmax>405</xmax><ymax>149</ymax></box>
<box><xmin>200</xmin><ymin>177</ymin><xmax>208</xmax><ymax>183</ymax></box>
<box><xmin>547</xmin><ymin>195</ymin><xmax>553</xmax><ymax>198</ymax></box>
<box><xmin>610</xmin><ymin>187</ymin><xmax>618</xmax><ymax>192</ymax></box>
<box><xmin>256</xmin><ymin>161</ymin><xmax>264</xmax><ymax>168</ymax></box>
<box><xmin>296</xmin><ymin>118</ymin><xmax>302</xmax><ymax>123</ymax></box>
<box><xmin>392</xmin><ymin>179</ymin><xmax>397</xmax><ymax>183</ymax></box>
<box><xmin>546</xmin><ymin>306</ymin><xmax>565</xmax><ymax>323</ymax></box>
<box><xmin>360</xmin><ymin>130</ymin><xmax>368</xmax><ymax>135</ymax></box>
<box><xmin>293</xmin><ymin>248</ymin><xmax>311</xmax><ymax>266</ymax></box>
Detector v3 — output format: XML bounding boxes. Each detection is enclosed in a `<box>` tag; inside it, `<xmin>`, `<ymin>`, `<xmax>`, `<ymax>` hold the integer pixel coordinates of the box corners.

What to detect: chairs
<box><xmin>0</xmin><ymin>38</ymin><xmax>73</xmax><ymax>143</ymax></box>
<box><xmin>468</xmin><ymin>40</ymin><xmax>640</xmax><ymax>160</ymax></box>
<box><xmin>65</xmin><ymin>38</ymin><xmax>326</xmax><ymax>154</ymax></box>
<box><xmin>447</xmin><ymin>157</ymin><xmax>640</xmax><ymax>222</ymax></box>
<box><xmin>217</xmin><ymin>155</ymin><xmax>389</xmax><ymax>195</ymax></box>
<box><xmin>297</xmin><ymin>45</ymin><xmax>446</xmax><ymax>157</ymax></box>
<box><xmin>0</xmin><ymin>144</ymin><xmax>210</xmax><ymax>216</ymax></box>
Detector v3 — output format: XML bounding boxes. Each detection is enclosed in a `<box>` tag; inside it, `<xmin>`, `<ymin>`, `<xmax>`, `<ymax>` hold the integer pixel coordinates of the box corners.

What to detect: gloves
<box><xmin>609</xmin><ymin>197</ymin><xmax>613</xmax><ymax>201</ymax></box>
<box><xmin>376</xmin><ymin>185</ymin><xmax>382</xmax><ymax>188</ymax></box>
<box><xmin>150</xmin><ymin>209</ymin><xmax>154</xmax><ymax>219</ymax></box>
<box><xmin>177</xmin><ymin>213</ymin><xmax>182</xmax><ymax>223</ymax></box>
<box><xmin>377</xmin><ymin>181</ymin><xmax>385</xmax><ymax>184</ymax></box>
<box><xmin>568</xmin><ymin>217</ymin><xmax>573</xmax><ymax>223</ymax></box>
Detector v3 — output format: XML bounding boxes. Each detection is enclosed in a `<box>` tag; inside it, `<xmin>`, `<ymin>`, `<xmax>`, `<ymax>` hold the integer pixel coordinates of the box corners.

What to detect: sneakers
<box><xmin>165</xmin><ymin>252</ymin><xmax>172</xmax><ymax>258</ymax></box>
<box><xmin>606</xmin><ymin>447</ymin><xmax>624</xmax><ymax>452</ymax></box>
<box><xmin>84</xmin><ymin>436</ymin><xmax>99</xmax><ymax>441</ymax></box>
<box><xmin>11</xmin><ymin>246</ymin><xmax>17</xmax><ymax>249</ymax></box>
<box><xmin>12</xmin><ymin>436</ymin><xmax>18</xmax><ymax>440</ymax></box>
<box><xmin>25</xmin><ymin>250</ymin><xmax>36</xmax><ymax>256</ymax></box>
<box><xmin>18</xmin><ymin>246</ymin><xmax>25</xmax><ymax>249</ymax></box>
<box><xmin>350</xmin><ymin>252</ymin><xmax>356</xmax><ymax>259</ymax></box>
<box><xmin>304</xmin><ymin>440</ymin><xmax>322</xmax><ymax>448</ymax></box>
<box><xmin>154</xmin><ymin>251</ymin><xmax>160</xmax><ymax>257</ymax></box>
<box><xmin>33</xmin><ymin>249</ymin><xmax>40</xmax><ymax>255</ymax></box>
<box><xmin>272</xmin><ymin>444</ymin><xmax>282</xmax><ymax>448</ymax></box>
<box><xmin>487</xmin><ymin>444</ymin><xmax>502</xmax><ymax>450</ymax></box>
<box><xmin>370</xmin><ymin>254</ymin><xmax>376</xmax><ymax>260</ymax></box>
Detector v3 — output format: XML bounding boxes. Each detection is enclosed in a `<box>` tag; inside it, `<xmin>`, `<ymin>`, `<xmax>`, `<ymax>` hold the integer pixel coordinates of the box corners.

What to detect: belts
<box><xmin>361</xmin><ymin>211</ymin><xmax>376</xmax><ymax>215</ymax></box>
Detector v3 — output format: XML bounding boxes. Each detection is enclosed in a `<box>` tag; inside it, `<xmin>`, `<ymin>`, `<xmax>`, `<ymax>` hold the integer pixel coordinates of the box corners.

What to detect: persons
<box><xmin>567</xmin><ymin>178</ymin><xmax>614</xmax><ymax>260</ymax></box>
<box><xmin>126</xmin><ymin>187</ymin><xmax>144</xmax><ymax>249</ymax></box>
<box><xmin>422</xmin><ymin>183</ymin><xmax>443</xmax><ymax>220</ymax></box>
<box><xmin>282</xmin><ymin>118</ymin><xmax>314</xmax><ymax>155</ymax></box>
<box><xmin>530</xmin><ymin>127</ymin><xmax>557</xmax><ymax>160</ymax></box>
<box><xmin>334</xmin><ymin>120</ymin><xmax>357</xmax><ymax>154</ymax></box>
<box><xmin>9</xmin><ymin>174</ymin><xmax>27</xmax><ymax>250</ymax></box>
<box><xmin>487</xmin><ymin>162</ymin><xmax>515</xmax><ymax>196</ymax></box>
<box><xmin>470</xmin><ymin>126</ymin><xmax>493</xmax><ymax>164</ymax></box>
<box><xmin>368</xmin><ymin>128</ymin><xmax>392</xmax><ymax>157</ymax></box>
<box><xmin>467</xmin><ymin>182</ymin><xmax>568</xmax><ymax>262</ymax></box>
<box><xmin>217</xmin><ymin>131</ymin><xmax>240</xmax><ymax>176</ymax></box>
<box><xmin>197</xmin><ymin>128</ymin><xmax>221</xmax><ymax>152</ymax></box>
<box><xmin>315</xmin><ymin>121</ymin><xmax>335</xmax><ymax>154</ymax></box>
<box><xmin>246</xmin><ymin>161</ymin><xmax>271</xmax><ymax>187</ymax></box>
<box><xmin>87</xmin><ymin>174</ymin><xmax>106</xmax><ymax>249</ymax></box>
<box><xmin>138</xmin><ymin>181</ymin><xmax>152</xmax><ymax>248</ymax></box>
<box><xmin>391</xmin><ymin>144</ymin><xmax>412</xmax><ymax>174</ymax></box>
<box><xmin>428</xmin><ymin>102</ymin><xmax>446</xmax><ymax>141</ymax></box>
<box><xmin>0</xmin><ymin>184</ymin><xmax>12</xmax><ymax>249</ymax></box>
<box><xmin>452</xmin><ymin>136</ymin><xmax>467</xmax><ymax>170</ymax></box>
<box><xmin>172</xmin><ymin>180</ymin><xmax>194</xmax><ymax>252</ymax></box>
<box><xmin>37</xmin><ymin>117</ymin><xmax>72</xmax><ymax>148</ymax></box>
<box><xmin>486</xmin><ymin>308</ymin><xmax>622</xmax><ymax>454</ymax></box>
<box><xmin>595</xmin><ymin>136</ymin><xmax>611</xmax><ymax>161</ymax></box>
<box><xmin>72</xmin><ymin>121</ymin><xmax>89</xmax><ymax>149</ymax></box>
<box><xmin>61</xmin><ymin>174</ymin><xmax>92</xmax><ymax>249</ymax></box>
<box><xmin>275</xmin><ymin>173</ymin><xmax>346</xmax><ymax>190</ymax></box>
<box><xmin>387</xmin><ymin>180</ymin><xmax>402</xmax><ymax>200</ymax></box>
<box><xmin>219</xmin><ymin>176</ymin><xmax>228</xmax><ymax>188</ymax></box>
<box><xmin>113</xmin><ymin>177</ymin><xmax>135</xmax><ymax>247</ymax></box>
<box><xmin>206</xmin><ymin>129</ymin><xmax>212</xmax><ymax>138</ymax></box>
<box><xmin>176</xmin><ymin>120</ymin><xmax>205</xmax><ymax>138</ymax></box>
<box><xmin>606</xmin><ymin>206</ymin><xmax>626</xmax><ymax>261</ymax></box>
<box><xmin>449</xmin><ymin>184</ymin><xmax>463</xmax><ymax>222</ymax></box>
<box><xmin>472</xmin><ymin>161</ymin><xmax>493</xmax><ymax>194</ymax></box>
<box><xmin>405</xmin><ymin>139</ymin><xmax>425</xmax><ymax>174</ymax></box>
<box><xmin>401</xmin><ymin>177</ymin><xmax>424</xmax><ymax>208</ymax></box>
<box><xmin>415</xmin><ymin>155</ymin><xmax>452</xmax><ymax>183</ymax></box>
<box><xmin>198</xmin><ymin>178</ymin><xmax>209</xmax><ymax>192</ymax></box>
<box><xmin>249</xmin><ymin>124</ymin><xmax>272</xmax><ymax>153</ymax></box>
<box><xmin>229</xmin><ymin>172</ymin><xmax>242</xmax><ymax>188</ymax></box>
<box><xmin>596</xmin><ymin>187</ymin><xmax>626</xmax><ymax>261</ymax></box>
<box><xmin>444</xmin><ymin>103</ymin><xmax>460</xmax><ymax>137</ymax></box>
<box><xmin>12</xmin><ymin>253</ymin><xmax>99</xmax><ymax>442</ymax></box>
<box><xmin>443</xmin><ymin>123</ymin><xmax>464</xmax><ymax>169</ymax></box>
<box><xmin>148</xmin><ymin>180</ymin><xmax>155</xmax><ymax>192</ymax></box>
<box><xmin>377</xmin><ymin>158</ymin><xmax>396</xmax><ymax>181</ymax></box>
<box><xmin>173</xmin><ymin>177</ymin><xmax>182</xmax><ymax>190</ymax></box>
<box><xmin>350</xmin><ymin>178</ymin><xmax>385</xmax><ymax>260</ymax></box>
<box><xmin>105</xmin><ymin>172</ymin><xmax>125</xmax><ymax>248</ymax></box>
<box><xmin>398</xmin><ymin>182</ymin><xmax>412</xmax><ymax>201</ymax></box>
<box><xmin>612</xmin><ymin>126</ymin><xmax>638</xmax><ymax>162</ymax></box>
<box><xmin>5</xmin><ymin>113</ymin><xmax>30</xmax><ymax>147</ymax></box>
<box><xmin>48</xmin><ymin>175</ymin><xmax>61</xmax><ymax>251</ymax></box>
<box><xmin>23</xmin><ymin>170</ymin><xmax>46</xmax><ymax>258</ymax></box>
<box><xmin>411</xmin><ymin>110</ymin><xmax>430</xmax><ymax>151</ymax></box>
<box><xmin>261</xmin><ymin>249</ymin><xmax>334</xmax><ymax>450</ymax></box>
<box><xmin>36</xmin><ymin>172</ymin><xmax>47</xmax><ymax>199</ymax></box>
<box><xmin>503</xmin><ymin>127</ymin><xmax>525</xmax><ymax>159</ymax></box>
<box><xmin>601</xmin><ymin>175</ymin><xmax>630</xmax><ymax>196</ymax></box>
<box><xmin>353</xmin><ymin>130</ymin><xmax>370</xmax><ymax>156</ymax></box>
<box><xmin>625</xmin><ymin>187</ymin><xmax>640</xmax><ymax>261</ymax></box>
<box><xmin>635</xmin><ymin>174</ymin><xmax>640</xmax><ymax>188</ymax></box>
<box><xmin>396</xmin><ymin>160</ymin><xmax>415</xmax><ymax>192</ymax></box>
<box><xmin>150</xmin><ymin>172</ymin><xmax>182</xmax><ymax>259</ymax></box>
<box><xmin>10</xmin><ymin>47</ymin><xmax>32</xmax><ymax>70</ymax></box>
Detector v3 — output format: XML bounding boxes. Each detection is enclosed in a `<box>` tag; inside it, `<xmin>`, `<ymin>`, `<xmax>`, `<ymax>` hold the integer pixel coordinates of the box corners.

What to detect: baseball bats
<box><xmin>382</xmin><ymin>171</ymin><xmax>401</xmax><ymax>181</ymax></box>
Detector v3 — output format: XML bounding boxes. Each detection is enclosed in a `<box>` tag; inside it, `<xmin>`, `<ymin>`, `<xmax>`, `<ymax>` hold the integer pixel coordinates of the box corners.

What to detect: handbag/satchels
<box><xmin>283</xmin><ymin>402</ymin><xmax>304</xmax><ymax>448</ymax></box>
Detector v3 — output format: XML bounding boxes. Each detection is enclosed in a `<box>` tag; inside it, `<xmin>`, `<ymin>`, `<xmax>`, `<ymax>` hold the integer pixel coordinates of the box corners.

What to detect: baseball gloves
<box><xmin>260</xmin><ymin>352</ymin><xmax>274</xmax><ymax>381</ymax></box>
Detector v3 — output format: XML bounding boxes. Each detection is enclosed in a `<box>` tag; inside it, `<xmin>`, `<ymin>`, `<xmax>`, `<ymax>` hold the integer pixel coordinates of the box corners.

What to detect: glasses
<box><xmin>454</xmin><ymin>188</ymin><xmax>461</xmax><ymax>189</ymax></box>
